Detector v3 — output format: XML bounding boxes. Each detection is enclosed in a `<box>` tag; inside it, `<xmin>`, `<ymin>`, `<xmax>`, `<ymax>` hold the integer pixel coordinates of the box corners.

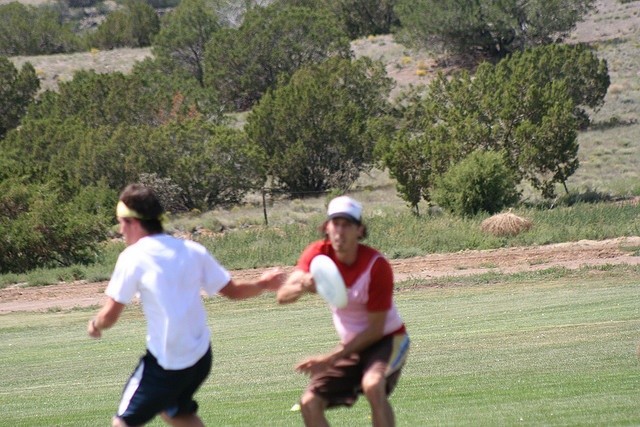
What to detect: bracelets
<box><xmin>92</xmin><ymin>319</ymin><xmax>98</xmax><ymax>331</ymax></box>
<box><xmin>338</xmin><ymin>342</ymin><xmax>351</xmax><ymax>360</ymax></box>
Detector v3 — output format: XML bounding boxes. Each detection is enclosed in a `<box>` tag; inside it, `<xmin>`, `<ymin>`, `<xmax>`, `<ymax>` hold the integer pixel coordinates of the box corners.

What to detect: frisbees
<box><xmin>309</xmin><ymin>254</ymin><xmax>348</xmax><ymax>309</ymax></box>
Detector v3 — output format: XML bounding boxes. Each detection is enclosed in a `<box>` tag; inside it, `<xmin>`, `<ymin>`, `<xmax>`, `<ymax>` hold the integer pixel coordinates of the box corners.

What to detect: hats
<box><xmin>327</xmin><ymin>195</ymin><xmax>362</xmax><ymax>225</ymax></box>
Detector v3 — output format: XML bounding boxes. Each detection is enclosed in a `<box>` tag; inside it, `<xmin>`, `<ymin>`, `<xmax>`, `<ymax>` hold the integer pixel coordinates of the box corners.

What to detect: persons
<box><xmin>87</xmin><ymin>186</ymin><xmax>291</xmax><ymax>427</ymax></box>
<box><xmin>277</xmin><ymin>196</ymin><xmax>412</xmax><ymax>427</ymax></box>
